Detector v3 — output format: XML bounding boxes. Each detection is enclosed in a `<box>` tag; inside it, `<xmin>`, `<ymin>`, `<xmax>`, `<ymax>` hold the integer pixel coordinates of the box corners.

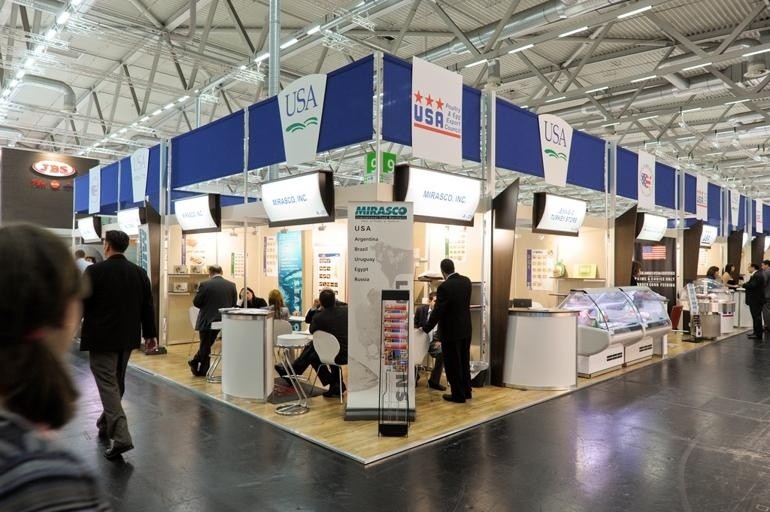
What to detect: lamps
<box><xmin>1</xmin><ymin>1</ymin><xmax>381</xmax><ymax>158</ymax></box>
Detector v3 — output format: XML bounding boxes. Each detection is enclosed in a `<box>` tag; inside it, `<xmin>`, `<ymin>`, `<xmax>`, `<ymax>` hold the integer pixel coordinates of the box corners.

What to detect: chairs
<box><xmin>188</xmin><ymin>307</ymin><xmax>208</xmax><ymax>356</ymax></box>
<box><xmin>308</xmin><ymin>329</ymin><xmax>346</xmax><ymax>405</ymax></box>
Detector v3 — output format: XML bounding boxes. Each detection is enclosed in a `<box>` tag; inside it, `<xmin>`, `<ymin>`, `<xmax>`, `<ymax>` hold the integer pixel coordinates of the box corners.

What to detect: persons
<box><xmin>631</xmin><ymin>262</ymin><xmax>642</xmax><ymax>285</ymax></box>
<box><xmin>75</xmin><ymin>251</ymin><xmax>94</xmax><ymax>338</ymax></box>
<box><xmin>706</xmin><ymin>258</ymin><xmax>770</xmax><ymax>339</ymax></box>
<box><xmin>268</xmin><ymin>289</ymin><xmax>296</xmax><ymax>366</ymax></box>
<box><xmin>76</xmin><ymin>231</ymin><xmax>162</xmax><ymax>457</ymax></box>
<box><xmin>85</xmin><ymin>254</ymin><xmax>96</xmax><ymax>266</ymax></box>
<box><xmin>239</xmin><ymin>287</ymin><xmax>267</xmax><ymax>308</ymax></box>
<box><xmin>274</xmin><ymin>299</ymin><xmax>348</xmax><ymax>386</ymax></box>
<box><xmin>2</xmin><ymin>225</ymin><xmax>112</xmax><ymax>510</ymax></box>
<box><xmin>416</xmin><ymin>260</ymin><xmax>472</xmax><ymax>405</ymax></box>
<box><xmin>415</xmin><ymin>291</ymin><xmax>447</xmax><ymax>391</ymax></box>
<box><xmin>311</xmin><ymin>288</ymin><xmax>348</xmax><ymax>397</ymax></box>
<box><xmin>187</xmin><ymin>266</ymin><xmax>237</xmax><ymax>377</ymax></box>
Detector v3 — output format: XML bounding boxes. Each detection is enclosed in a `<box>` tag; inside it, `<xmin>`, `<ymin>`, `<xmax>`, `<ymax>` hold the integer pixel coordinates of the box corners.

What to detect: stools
<box><xmin>274</xmin><ymin>334</ymin><xmax>312</xmax><ymax>415</ymax></box>
<box><xmin>206</xmin><ymin>321</ymin><xmax>224</xmax><ymax>383</ymax></box>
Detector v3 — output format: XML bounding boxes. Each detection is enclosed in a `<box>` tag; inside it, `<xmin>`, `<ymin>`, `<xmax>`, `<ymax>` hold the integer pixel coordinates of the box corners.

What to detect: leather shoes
<box><xmin>428</xmin><ymin>384</ymin><xmax>446</xmax><ymax>391</ymax></box>
<box><xmin>443</xmin><ymin>393</ymin><xmax>472</xmax><ymax>403</ymax></box>
<box><xmin>747</xmin><ymin>332</ymin><xmax>762</xmax><ymax>339</ymax></box>
<box><xmin>103</xmin><ymin>444</ymin><xmax>133</xmax><ymax>459</ymax></box>
<box><xmin>322</xmin><ymin>382</ymin><xmax>346</xmax><ymax>397</ymax></box>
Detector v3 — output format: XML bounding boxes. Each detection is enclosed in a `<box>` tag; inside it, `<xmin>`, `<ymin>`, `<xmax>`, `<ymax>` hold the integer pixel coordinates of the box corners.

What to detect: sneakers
<box><xmin>275</xmin><ymin>365</ymin><xmax>296</xmax><ymax>387</ymax></box>
<box><xmin>188</xmin><ymin>359</ymin><xmax>199</xmax><ymax>376</ymax></box>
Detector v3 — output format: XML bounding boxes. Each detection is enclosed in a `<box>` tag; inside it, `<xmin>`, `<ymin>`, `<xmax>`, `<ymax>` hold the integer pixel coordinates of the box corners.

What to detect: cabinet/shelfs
<box><xmin>167</xmin><ymin>269</ymin><xmax>211</xmax><ymax>295</ymax></box>
<box><xmin>559</xmin><ymin>288</ymin><xmax>668</xmax><ymax>337</ymax></box>
